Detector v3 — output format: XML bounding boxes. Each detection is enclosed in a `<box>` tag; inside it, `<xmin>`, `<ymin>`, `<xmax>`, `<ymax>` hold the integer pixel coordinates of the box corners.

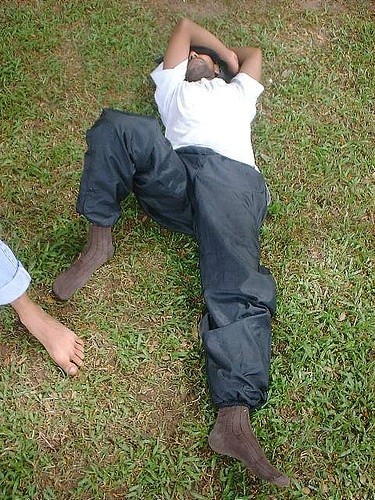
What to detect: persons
<box><xmin>50</xmin><ymin>17</ymin><xmax>291</xmax><ymax>490</ymax></box>
<box><xmin>0</xmin><ymin>240</ymin><xmax>85</xmax><ymax>377</ymax></box>
